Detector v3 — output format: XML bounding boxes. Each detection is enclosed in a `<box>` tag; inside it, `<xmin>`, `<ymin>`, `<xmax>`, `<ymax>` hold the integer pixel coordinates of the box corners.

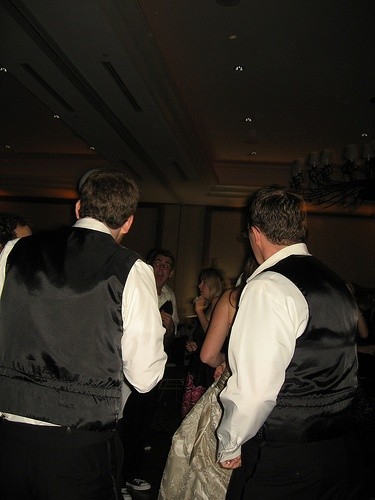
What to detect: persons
<box><xmin>0</xmin><ymin>215</ymin><xmax>33</xmax><ymax>254</ymax></box>
<box><xmin>216</xmin><ymin>182</ymin><xmax>375</xmax><ymax>500</ymax></box>
<box><xmin>153</xmin><ymin>228</ymin><xmax>312</xmax><ymax>500</ymax></box>
<box><xmin>0</xmin><ymin>167</ymin><xmax>168</xmax><ymax>500</ymax></box>
<box><xmin>182</xmin><ymin>267</ymin><xmax>227</xmax><ymax>416</ymax></box>
<box><xmin>118</xmin><ymin>249</ymin><xmax>179</xmax><ymax>500</ymax></box>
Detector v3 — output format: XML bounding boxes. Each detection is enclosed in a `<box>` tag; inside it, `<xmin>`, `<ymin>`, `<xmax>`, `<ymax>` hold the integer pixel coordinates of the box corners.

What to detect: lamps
<box><xmin>288</xmin><ymin>140</ymin><xmax>375</xmax><ymax>214</ymax></box>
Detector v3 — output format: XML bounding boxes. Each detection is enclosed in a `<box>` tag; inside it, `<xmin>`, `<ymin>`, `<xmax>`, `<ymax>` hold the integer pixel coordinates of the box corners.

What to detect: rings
<box><xmin>196</xmin><ymin>296</ymin><xmax>198</xmax><ymax>299</ymax></box>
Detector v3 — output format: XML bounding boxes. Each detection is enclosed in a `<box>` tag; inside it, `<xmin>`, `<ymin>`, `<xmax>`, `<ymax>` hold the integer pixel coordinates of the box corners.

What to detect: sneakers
<box><xmin>126</xmin><ymin>476</ymin><xmax>151</xmax><ymax>491</ymax></box>
<box><xmin>120</xmin><ymin>488</ymin><xmax>132</xmax><ymax>500</ymax></box>
